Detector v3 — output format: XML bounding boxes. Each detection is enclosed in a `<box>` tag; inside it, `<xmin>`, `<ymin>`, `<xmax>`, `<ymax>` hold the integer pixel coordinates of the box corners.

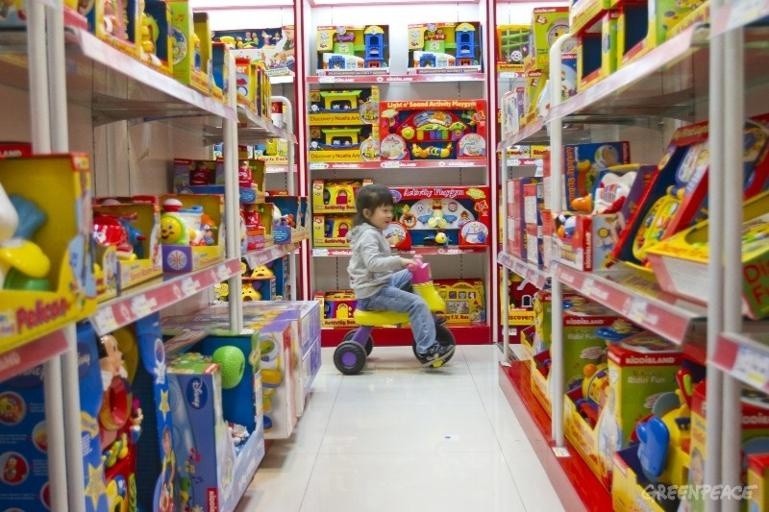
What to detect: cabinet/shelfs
<box><xmin>551</xmin><ymin>33</ymin><xmax>722</xmax><ymax>510</ymax></box>
<box><xmin>502</xmin><ymin>88</ymin><xmax>551</xmax><ymax>404</ymax></box>
<box><xmin>714</xmin><ymin>10</ymin><xmax>767</xmax><ymax>511</ymax></box>
<box><xmin>2</xmin><ymin>9</ymin><xmax>74</xmax><ymax>512</ymax></box>
<box><xmin>56</xmin><ymin>2</ymin><xmax>237</xmax><ymax>509</ymax></box>
<box><xmin>227</xmin><ymin>60</ymin><xmax>310</xmax><ymax>434</ymax></box>
<box><xmin>303</xmin><ymin>5</ymin><xmax>495</xmax><ymax>341</ymax></box>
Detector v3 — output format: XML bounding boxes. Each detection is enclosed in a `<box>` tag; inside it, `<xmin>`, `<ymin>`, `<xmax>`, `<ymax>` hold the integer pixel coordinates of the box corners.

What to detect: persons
<box><xmin>485</xmin><ymin>2</ymin><xmax>769</xmax><ymax>512</ymax></box>
<box><xmin>346</xmin><ymin>182</ymin><xmax>456</xmax><ymax>368</ymax></box>
<box><xmin>430</xmin><ymin>277</ymin><xmax>487</xmax><ymax>326</ymax></box>
<box><xmin>313</xmin><ymin>288</ymin><xmax>358</xmax><ymax>328</ymax></box>
<box><xmin>306</xmin><ymin>21</ymin><xmax>494</xmax><ymax>251</ymax></box>
<box><xmin>0</xmin><ymin>2</ymin><xmax>306</xmax><ymax>511</ymax></box>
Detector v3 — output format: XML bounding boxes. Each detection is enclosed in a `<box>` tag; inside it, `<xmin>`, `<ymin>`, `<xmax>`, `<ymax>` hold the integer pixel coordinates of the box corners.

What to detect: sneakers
<box><xmin>420</xmin><ymin>344</ymin><xmax>455</xmax><ymax>369</ymax></box>
